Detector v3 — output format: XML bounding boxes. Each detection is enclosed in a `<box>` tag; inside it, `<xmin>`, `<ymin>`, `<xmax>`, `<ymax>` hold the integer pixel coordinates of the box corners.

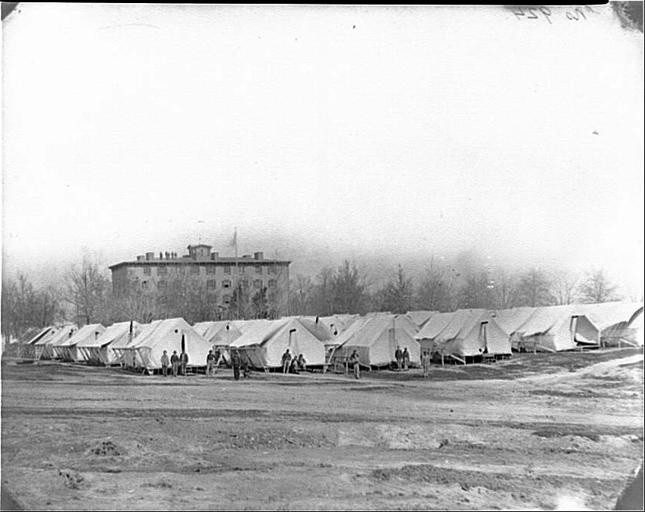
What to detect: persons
<box><xmin>422</xmin><ymin>351</ymin><xmax>430</xmax><ymax>376</ymax></box>
<box><xmin>171</xmin><ymin>351</ymin><xmax>179</xmax><ymax>377</ymax></box>
<box><xmin>233</xmin><ymin>353</ymin><xmax>240</xmax><ymax>381</ymax></box>
<box><xmin>206</xmin><ymin>350</ymin><xmax>217</xmax><ymax>374</ymax></box>
<box><xmin>350</xmin><ymin>350</ymin><xmax>360</xmax><ymax>379</ymax></box>
<box><xmin>180</xmin><ymin>351</ymin><xmax>189</xmax><ymax>375</ymax></box>
<box><xmin>161</xmin><ymin>350</ymin><xmax>169</xmax><ymax>376</ymax></box>
<box><xmin>282</xmin><ymin>349</ymin><xmax>292</xmax><ymax>373</ymax></box>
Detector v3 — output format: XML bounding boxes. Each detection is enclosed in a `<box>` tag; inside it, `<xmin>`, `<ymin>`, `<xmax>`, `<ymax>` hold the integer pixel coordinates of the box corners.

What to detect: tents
<box><xmin>26</xmin><ymin>299</ymin><xmax>645</xmax><ymax>371</ymax></box>
